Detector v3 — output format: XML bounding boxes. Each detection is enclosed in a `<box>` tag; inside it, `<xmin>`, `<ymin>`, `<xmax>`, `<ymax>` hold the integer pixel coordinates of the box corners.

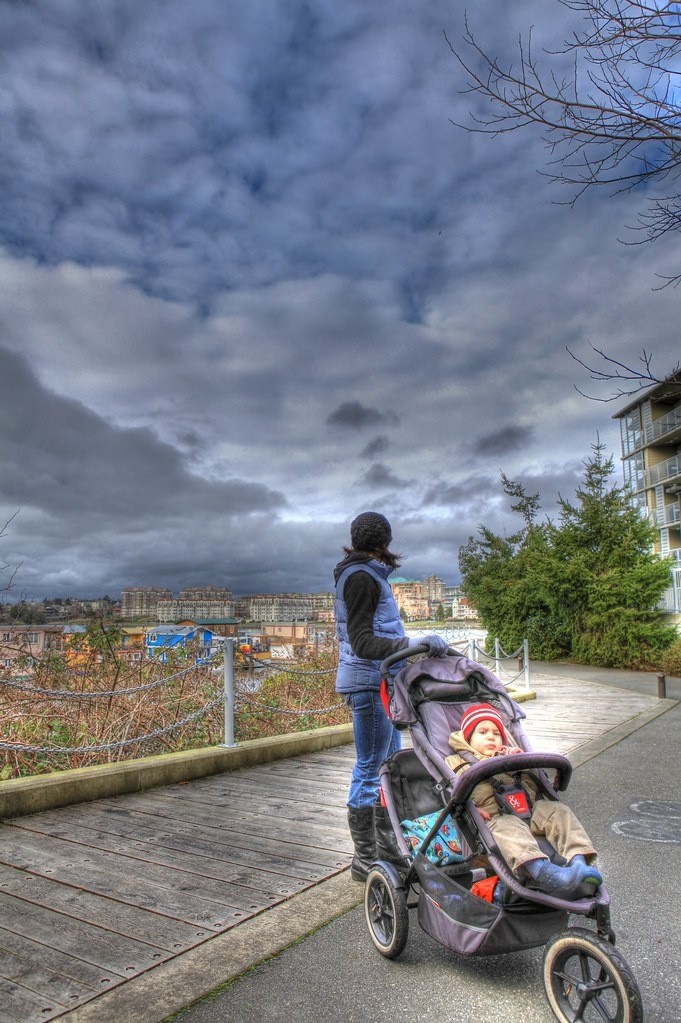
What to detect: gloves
<box><xmin>408</xmin><ymin>634</ymin><xmax>449</xmax><ymax>658</ymax></box>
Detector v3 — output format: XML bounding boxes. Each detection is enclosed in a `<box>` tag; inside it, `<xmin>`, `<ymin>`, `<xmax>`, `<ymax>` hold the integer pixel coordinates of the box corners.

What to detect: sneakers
<box><xmin>566</xmin><ymin>855</ymin><xmax>603</xmax><ymax>886</ymax></box>
<box><xmin>526</xmin><ymin>861</ymin><xmax>585</xmax><ymax>897</ymax></box>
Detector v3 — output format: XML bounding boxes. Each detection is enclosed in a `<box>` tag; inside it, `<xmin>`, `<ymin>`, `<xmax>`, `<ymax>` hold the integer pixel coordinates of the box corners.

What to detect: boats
<box><xmin>234</xmin><ymin>643</ymin><xmax>274</xmax><ymax>669</ymax></box>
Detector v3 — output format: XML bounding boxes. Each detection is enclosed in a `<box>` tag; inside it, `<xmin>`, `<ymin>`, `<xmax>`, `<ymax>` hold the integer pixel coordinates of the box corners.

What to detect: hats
<box><xmin>350</xmin><ymin>512</ymin><xmax>393</xmax><ymax>550</ymax></box>
<box><xmin>461</xmin><ymin>703</ymin><xmax>509</xmax><ymax>746</ymax></box>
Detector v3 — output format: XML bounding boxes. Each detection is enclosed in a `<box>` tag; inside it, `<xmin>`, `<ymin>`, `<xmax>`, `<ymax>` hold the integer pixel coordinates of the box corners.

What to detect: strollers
<box><xmin>364</xmin><ymin>643</ymin><xmax>644</xmax><ymax>1023</ymax></box>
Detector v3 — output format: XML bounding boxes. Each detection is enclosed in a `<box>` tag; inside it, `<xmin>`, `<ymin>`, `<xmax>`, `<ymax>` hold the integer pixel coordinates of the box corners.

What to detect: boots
<box><xmin>346</xmin><ymin>804</ymin><xmax>405</xmax><ymax>883</ymax></box>
<box><xmin>374</xmin><ymin>798</ymin><xmax>419</xmax><ymax>883</ymax></box>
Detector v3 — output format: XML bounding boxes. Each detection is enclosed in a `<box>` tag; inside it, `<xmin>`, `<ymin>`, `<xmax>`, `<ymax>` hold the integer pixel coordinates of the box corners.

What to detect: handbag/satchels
<box><xmin>400</xmin><ymin>808</ymin><xmax>464</xmax><ymax>866</ymax></box>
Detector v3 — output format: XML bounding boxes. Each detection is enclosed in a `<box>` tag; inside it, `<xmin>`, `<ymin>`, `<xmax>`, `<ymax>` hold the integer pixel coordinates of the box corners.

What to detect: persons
<box><xmin>334</xmin><ymin>512</ymin><xmax>450</xmax><ymax>883</ymax></box>
<box><xmin>446</xmin><ymin>703</ymin><xmax>604</xmax><ymax>900</ymax></box>
